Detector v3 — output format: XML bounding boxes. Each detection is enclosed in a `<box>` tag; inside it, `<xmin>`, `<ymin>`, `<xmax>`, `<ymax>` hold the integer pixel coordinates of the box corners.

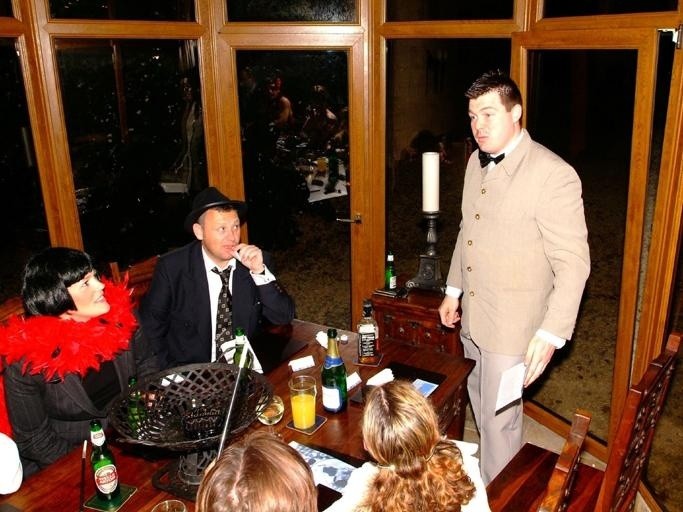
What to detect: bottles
<box><xmin>321</xmin><ymin>327</ymin><xmax>347</xmax><ymax>414</ymax></box>
<box><xmin>233</xmin><ymin>325</ymin><xmax>249</xmax><ymax>369</ymax></box>
<box><xmin>329</xmin><ymin>141</ymin><xmax>338</xmax><ymax>183</ymax></box>
<box><xmin>124</xmin><ymin>375</ymin><xmax>146</xmax><ymax>445</ymax></box>
<box><xmin>357</xmin><ymin>301</ymin><xmax>380</xmax><ymax>365</ymax></box>
<box><xmin>383</xmin><ymin>251</ymin><xmax>397</xmax><ymax>290</ymax></box>
<box><xmin>89</xmin><ymin>419</ymin><xmax>119</xmax><ymax>500</ymax></box>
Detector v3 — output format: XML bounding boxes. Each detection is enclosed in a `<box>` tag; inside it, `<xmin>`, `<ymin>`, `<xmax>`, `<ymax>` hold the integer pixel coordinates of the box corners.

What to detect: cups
<box><xmin>316</xmin><ymin>156</ymin><xmax>327</xmax><ymax>175</ymax></box>
<box><xmin>287</xmin><ymin>375</ymin><xmax>317</xmax><ymax>429</ymax></box>
<box><xmin>150</xmin><ymin>500</ymin><xmax>186</xmax><ymax>512</ymax></box>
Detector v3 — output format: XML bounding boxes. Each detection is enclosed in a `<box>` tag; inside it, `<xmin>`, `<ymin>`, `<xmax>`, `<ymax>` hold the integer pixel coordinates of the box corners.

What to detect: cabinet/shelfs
<box><xmin>366</xmin><ymin>286</ymin><xmax>465</xmax><ymax>361</ymax></box>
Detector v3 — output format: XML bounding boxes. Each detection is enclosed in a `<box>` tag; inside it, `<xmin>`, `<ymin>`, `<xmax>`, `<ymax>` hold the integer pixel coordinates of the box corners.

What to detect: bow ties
<box><xmin>478</xmin><ymin>153</ymin><xmax>505</xmax><ymax>169</ymax></box>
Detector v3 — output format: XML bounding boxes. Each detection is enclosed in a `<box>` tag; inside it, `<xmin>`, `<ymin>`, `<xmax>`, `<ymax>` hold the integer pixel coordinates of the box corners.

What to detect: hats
<box><xmin>185</xmin><ymin>187</ymin><xmax>249</xmax><ymax>229</ymax></box>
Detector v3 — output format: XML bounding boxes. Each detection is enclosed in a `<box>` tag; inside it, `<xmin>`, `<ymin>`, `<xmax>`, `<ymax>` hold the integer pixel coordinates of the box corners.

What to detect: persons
<box><xmin>172</xmin><ymin>65</ymin><xmax>208</xmax><ymax>197</ymax></box>
<box><xmin>439</xmin><ymin>68</ymin><xmax>591</xmax><ymax>489</ymax></box>
<box><xmin>195</xmin><ymin>432</ymin><xmax>318</xmax><ymax>511</ymax></box>
<box><xmin>137</xmin><ymin>187</ymin><xmax>296</xmax><ymax>376</ymax></box>
<box><xmin>0</xmin><ymin>247</ymin><xmax>161</xmax><ymax>480</ymax></box>
<box><xmin>236</xmin><ymin>66</ymin><xmax>350</xmax><ymax>252</ymax></box>
<box><xmin>319</xmin><ymin>381</ymin><xmax>494</xmax><ymax>511</ymax></box>
<box><xmin>397</xmin><ymin>130</ymin><xmax>457</xmax><ymax>188</ymax></box>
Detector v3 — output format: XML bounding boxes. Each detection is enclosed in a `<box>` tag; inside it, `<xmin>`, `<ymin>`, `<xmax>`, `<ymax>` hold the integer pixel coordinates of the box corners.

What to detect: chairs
<box><xmin>487</xmin><ymin>332</ymin><xmax>682</xmax><ymax>511</ymax></box>
<box><xmin>109</xmin><ymin>260</ymin><xmax>155</xmax><ymax>316</ymax></box>
<box><xmin>538</xmin><ymin>406</ymin><xmax>590</xmax><ymax>510</ymax></box>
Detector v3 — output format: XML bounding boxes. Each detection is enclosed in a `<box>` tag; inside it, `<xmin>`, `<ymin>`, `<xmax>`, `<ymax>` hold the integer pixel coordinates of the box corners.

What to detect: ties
<box><xmin>211</xmin><ymin>265</ymin><xmax>234</xmax><ymax>362</ymax></box>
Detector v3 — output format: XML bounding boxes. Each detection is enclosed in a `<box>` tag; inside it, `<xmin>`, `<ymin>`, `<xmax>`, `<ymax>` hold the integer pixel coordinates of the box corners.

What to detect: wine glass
<box><xmin>256</xmin><ymin>394</ymin><xmax>285</xmax><ymax>445</ymax></box>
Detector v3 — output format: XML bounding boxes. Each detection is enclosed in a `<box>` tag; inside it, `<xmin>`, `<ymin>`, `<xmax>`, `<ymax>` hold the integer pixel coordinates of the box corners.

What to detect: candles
<box><xmin>420</xmin><ymin>152</ymin><xmax>441</xmax><ymax>212</ymax></box>
